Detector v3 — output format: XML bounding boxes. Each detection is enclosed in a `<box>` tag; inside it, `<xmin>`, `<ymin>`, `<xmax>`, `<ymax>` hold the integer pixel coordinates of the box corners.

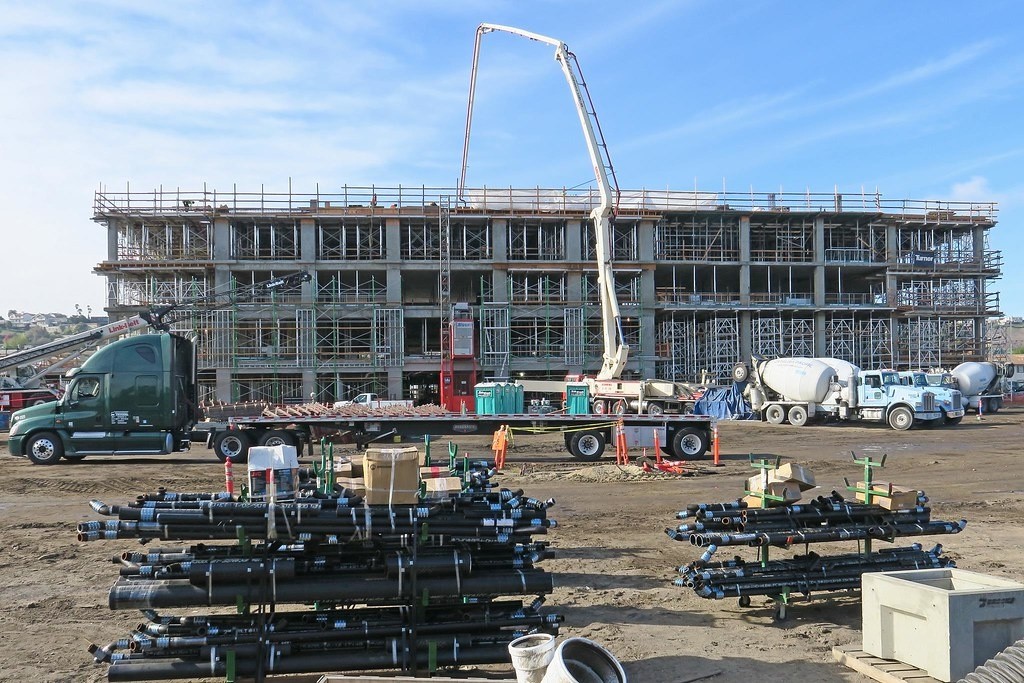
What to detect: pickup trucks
<box><xmin>333</xmin><ymin>392</ymin><xmax>415</xmax><ymax>409</ymax></box>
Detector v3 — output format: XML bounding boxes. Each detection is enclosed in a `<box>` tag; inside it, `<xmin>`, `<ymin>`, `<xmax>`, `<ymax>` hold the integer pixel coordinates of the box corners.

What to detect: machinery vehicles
<box><xmin>456</xmin><ymin>22</ymin><xmax>698</xmax><ymax>415</ymax></box>
<box><xmin>0</xmin><ymin>266</ymin><xmax>1007</xmax><ymax>466</ymax></box>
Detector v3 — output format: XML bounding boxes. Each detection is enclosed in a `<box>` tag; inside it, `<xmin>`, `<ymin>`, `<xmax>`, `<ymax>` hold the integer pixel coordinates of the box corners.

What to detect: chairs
<box><xmin>92</xmin><ymin>383</ymin><xmax>99</xmax><ymax>396</ymax></box>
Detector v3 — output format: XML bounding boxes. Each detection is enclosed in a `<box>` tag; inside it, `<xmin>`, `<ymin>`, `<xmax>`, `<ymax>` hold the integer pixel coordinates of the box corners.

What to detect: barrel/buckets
<box><xmin>508</xmin><ymin>633</ymin><xmax>555</xmax><ymax>683</ymax></box>
<box><xmin>541</xmin><ymin>637</ymin><xmax>627</xmax><ymax>683</ymax></box>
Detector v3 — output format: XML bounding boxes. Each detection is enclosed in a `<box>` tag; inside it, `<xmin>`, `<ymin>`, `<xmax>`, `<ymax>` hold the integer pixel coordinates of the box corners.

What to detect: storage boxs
<box><xmin>248</xmin><ymin>444</ymin><xmax>299</xmax><ymax>501</ymax></box>
<box><xmin>741</xmin><ymin>463</ymin><xmax>816</xmax><ymax>508</ymax></box>
<box><xmin>362</xmin><ymin>446</ymin><xmax>419</xmax><ymax>504</ymax></box>
<box><xmin>862</xmin><ymin>568</ymin><xmax>1024</xmax><ymax>683</ymax></box>
<box><xmin>856</xmin><ymin>480</ymin><xmax>917</xmax><ymax>509</ymax></box>
<box><xmin>325</xmin><ymin>455</ymin><xmax>462</xmax><ymax>496</ymax></box>
